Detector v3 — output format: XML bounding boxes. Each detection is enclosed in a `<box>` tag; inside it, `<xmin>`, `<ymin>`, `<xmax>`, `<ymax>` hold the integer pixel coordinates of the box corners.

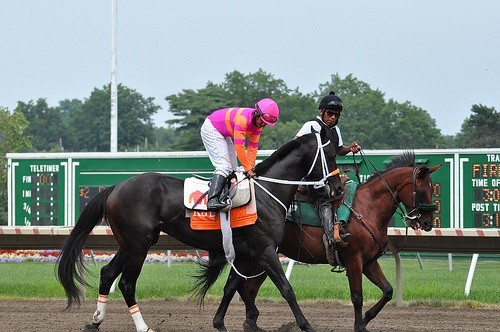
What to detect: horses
<box><xmin>242</xmin><ymin>148</ymin><xmax>442</xmax><ymax>332</ymax></box>
<box><xmin>53</xmin><ymin>125</ymin><xmax>344</xmax><ymax>332</ymax></box>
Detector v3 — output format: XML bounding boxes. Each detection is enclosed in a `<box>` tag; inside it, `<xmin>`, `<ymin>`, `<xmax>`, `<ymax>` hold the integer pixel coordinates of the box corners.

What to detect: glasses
<box><xmin>324</xmin><ymin>109</ymin><xmax>340</xmax><ymax>118</ymax></box>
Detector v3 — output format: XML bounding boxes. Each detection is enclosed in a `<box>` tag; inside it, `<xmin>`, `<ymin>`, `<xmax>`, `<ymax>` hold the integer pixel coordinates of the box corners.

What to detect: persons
<box><xmin>201</xmin><ymin>98</ymin><xmax>279</xmax><ymax>211</ymax></box>
<box><xmin>292</xmin><ymin>91</ymin><xmax>361</xmax><ymax>249</ymax></box>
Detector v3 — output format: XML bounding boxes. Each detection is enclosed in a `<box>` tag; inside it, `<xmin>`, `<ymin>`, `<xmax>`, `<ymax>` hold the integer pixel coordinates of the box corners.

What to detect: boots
<box><xmin>207</xmin><ymin>174</ymin><xmax>231</xmax><ymax>211</ymax></box>
<box><xmin>312</xmin><ymin>197</ymin><xmax>352</xmax><ymax>246</ymax></box>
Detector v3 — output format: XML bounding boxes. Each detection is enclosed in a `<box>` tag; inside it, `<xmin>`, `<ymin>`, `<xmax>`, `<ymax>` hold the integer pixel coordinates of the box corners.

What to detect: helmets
<box><xmin>318</xmin><ymin>91</ymin><xmax>344</xmax><ymax>110</ymax></box>
<box><xmin>255</xmin><ymin>97</ymin><xmax>279</xmax><ymax>126</ymax></box>
